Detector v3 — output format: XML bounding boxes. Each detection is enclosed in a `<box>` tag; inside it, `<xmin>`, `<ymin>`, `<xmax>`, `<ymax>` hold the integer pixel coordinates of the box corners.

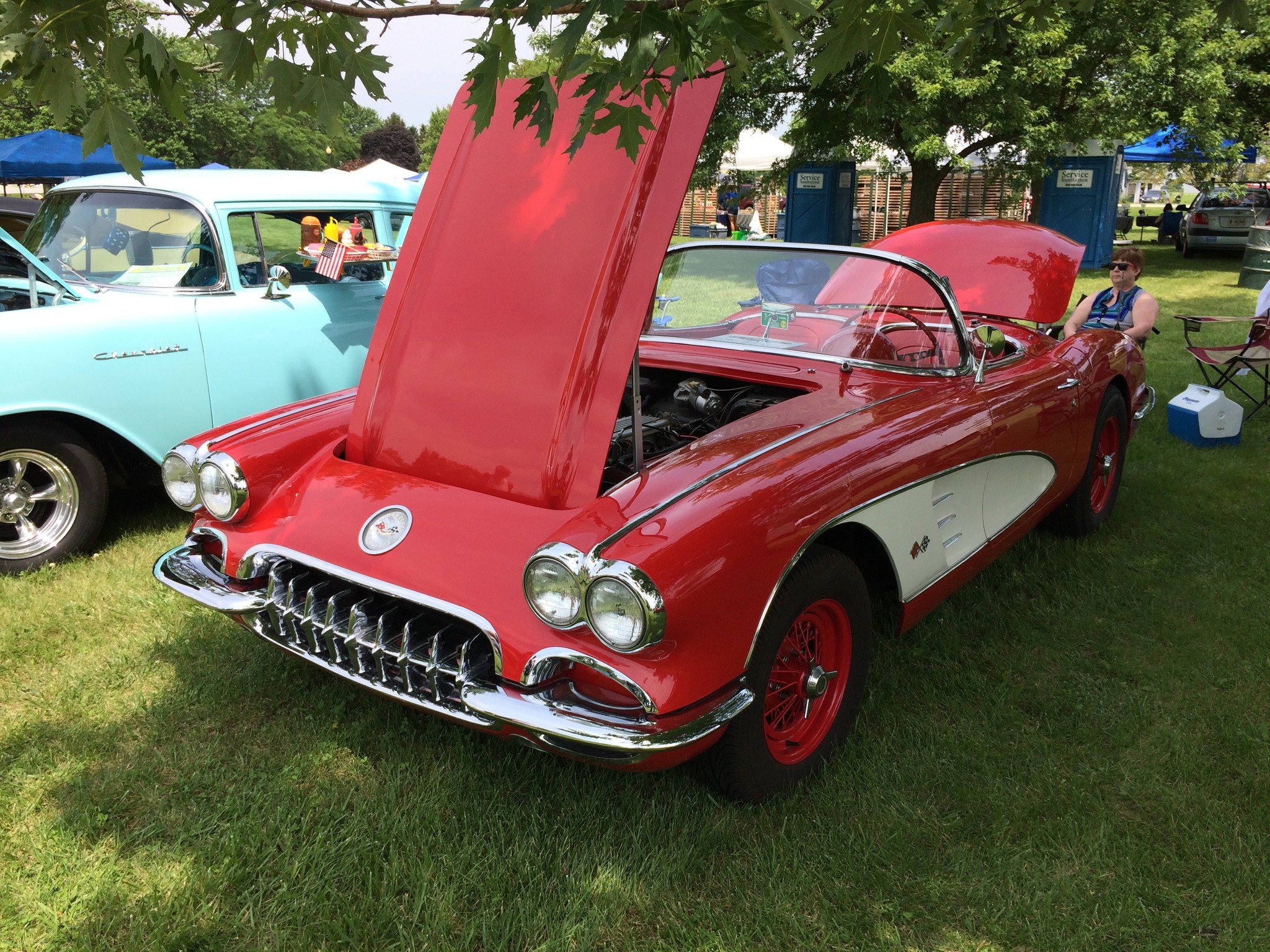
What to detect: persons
<box><xmin>1242</xmin><ymin>188</ymin><xmax>1259</xmax><ymax>204</ymax></box>
<box><xmin>717</xmin><ymin>181</ymin><xmax>741</xmax><ymax>237</ymax></box>
<box><xmin>1154</xmin><ymin>203</ymin><xmax>1174</xmax><ymax>233</ymax></box>
<box><xmin>1061</xmin><ymin>247</ymin><xmax>1159</xmax><ymax>350</ymax></box>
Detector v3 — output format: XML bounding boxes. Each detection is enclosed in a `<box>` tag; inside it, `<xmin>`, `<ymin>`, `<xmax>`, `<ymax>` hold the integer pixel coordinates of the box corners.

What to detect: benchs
<box><xmin>196</xmin><ymin>263</ymin><xmax>328</xmax><ymax>290</ymax></box>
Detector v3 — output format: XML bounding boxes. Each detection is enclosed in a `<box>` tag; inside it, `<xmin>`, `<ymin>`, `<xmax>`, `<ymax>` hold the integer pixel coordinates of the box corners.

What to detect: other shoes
<box><xmin>730</xmin><ymin>235</ymin><xmax>733</xmax><ymax>238</ymax></box>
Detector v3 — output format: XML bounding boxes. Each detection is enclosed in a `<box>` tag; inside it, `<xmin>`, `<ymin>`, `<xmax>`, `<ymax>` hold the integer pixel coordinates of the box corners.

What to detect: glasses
<box><xmin>1106</xmin><ymin>262</ymin><xmax>1136</xmax><ymax>271</ymax></box>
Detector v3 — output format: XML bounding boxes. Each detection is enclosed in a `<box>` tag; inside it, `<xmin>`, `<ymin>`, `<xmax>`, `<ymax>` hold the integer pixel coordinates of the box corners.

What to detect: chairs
<box><xmin>652</xmin><ymin>273</ymin><xmax>680</xmax><ymax>326</ymax></box>
<box><xmin>1160</xmin><ymin>211</ymin><xmax>1183</xmax><ymax>244</ymax></box>
<box><xmin>857</xmin><ymin>316</ymin><xmax>1003</xmax><ymax>371</ymax></box>
<box><xmin>731</xmin><ymin>312</ymin><xmax>857</xmax><ymax>366</ymax></box>
<box><xmin>738</xmin><ymin>258</ymin><xmax>831</xmax><ymax>308</ymax></box>
<box><xmin>1173</xmin><ymin>307</ymin><xmax>1270</xmax><ymax>415</ymax></box>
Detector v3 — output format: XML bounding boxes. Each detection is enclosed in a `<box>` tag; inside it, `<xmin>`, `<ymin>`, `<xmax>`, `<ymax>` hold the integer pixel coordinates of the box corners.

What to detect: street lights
<box><xmin>326</xmin><ymin>146</ymin><xmax>331</xmax><ymax>169</ymax></box>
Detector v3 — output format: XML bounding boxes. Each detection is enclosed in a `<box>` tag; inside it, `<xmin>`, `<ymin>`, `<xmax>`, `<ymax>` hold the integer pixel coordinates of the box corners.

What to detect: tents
<box><xmin>1121</xmin><ymin>122</ymin><xmax>1257</xmax><ymax>182</ymax></box>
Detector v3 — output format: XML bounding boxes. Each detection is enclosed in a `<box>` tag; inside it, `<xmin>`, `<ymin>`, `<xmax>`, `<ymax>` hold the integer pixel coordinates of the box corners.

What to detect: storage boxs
<box><xmin>732</xmin><ymin>231</ymin><xmax>746</xmax><ymax>241</ymax></box>
<box><xmin>709</xmin><ymin>227</ymin><xmax>728</xmax><ymax>238</ymax></box>
<box><xmin>690</xmin><ymin>224</ymin><xmax>709</xmax><ymax>238</ymax></box>
<box><xmin>776</xmin><ymin>206</ymin><xmax>862</xmax><ymax>243</ymax></box>
<box><xmin>1166</xmin><ymin>383</ymin><xmax>1246</xmax><ymax>446</ymax></box>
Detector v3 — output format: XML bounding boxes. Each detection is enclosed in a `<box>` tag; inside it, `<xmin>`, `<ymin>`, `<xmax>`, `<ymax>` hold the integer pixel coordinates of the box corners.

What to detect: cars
<box><xmin>0</xmin><ymin>168</ymin><xmax>419</xmax><ymax>578</ymax></box>
<box><xmin>152</xmin><ymin>60</ymin><xmax>1156</xmax><ymax>801</ymax></box>
<box><xmin>1175</xmin><ymin>177</ymin><xmax>1270</xmax><ymax>258</ymax></box>
<box><xmin>1140</xmin><ymin>189</ymin><xmax>1171</xmax><ymax>204</ymax></box>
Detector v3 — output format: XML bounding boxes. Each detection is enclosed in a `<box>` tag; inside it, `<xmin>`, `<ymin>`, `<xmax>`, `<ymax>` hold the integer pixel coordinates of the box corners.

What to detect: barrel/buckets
<box><xmin>731</xmin><ymin>230</ymin><xmax>749</xmax><ymax>241</ymax></box>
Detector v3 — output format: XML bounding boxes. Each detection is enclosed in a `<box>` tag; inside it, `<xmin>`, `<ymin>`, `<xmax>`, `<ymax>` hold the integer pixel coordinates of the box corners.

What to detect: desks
<box><xmin>715</xmin><ymin>209</ymin><xmax>753</xmax><ymax>235</ymax></box>
<box><xmin>1136</xmin><ymin>216</ymin><xmax>1159</xmax><ymax>243</ymax></box>
<box><xmin>1116</xmin><ymin>216</ymin><xmax>1134</xmax><ymax>241</ymax></box>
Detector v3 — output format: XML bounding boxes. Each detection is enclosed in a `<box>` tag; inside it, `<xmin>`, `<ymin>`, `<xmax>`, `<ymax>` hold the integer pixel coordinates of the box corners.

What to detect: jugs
<box><xmin>301</xmin><ymin>216</ymin><xmax>325</xmax><ymax>254</ymax></box>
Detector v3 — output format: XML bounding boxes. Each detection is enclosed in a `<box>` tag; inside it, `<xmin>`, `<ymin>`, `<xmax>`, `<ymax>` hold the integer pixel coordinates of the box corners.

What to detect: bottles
<box><xmin>349</xmin><ymin>217</ymin><xmax>362</xmax><ymax>242</ymax></box>
<box><xmin>324</xmin><ymin>216</ymin><xmax>339</xmax><ymax>245</ymax></box>
<box><xmin>658</xmin><ymin>294</ymin><xmax>668</xmax><ymax>311</ymax></box>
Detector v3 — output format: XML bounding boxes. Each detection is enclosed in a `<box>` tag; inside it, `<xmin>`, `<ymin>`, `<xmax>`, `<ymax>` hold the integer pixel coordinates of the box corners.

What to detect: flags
<box><xmin>315</xmin><ymin>237</ymin><xmax>350</xmax><ymax>281</ymax></box>
